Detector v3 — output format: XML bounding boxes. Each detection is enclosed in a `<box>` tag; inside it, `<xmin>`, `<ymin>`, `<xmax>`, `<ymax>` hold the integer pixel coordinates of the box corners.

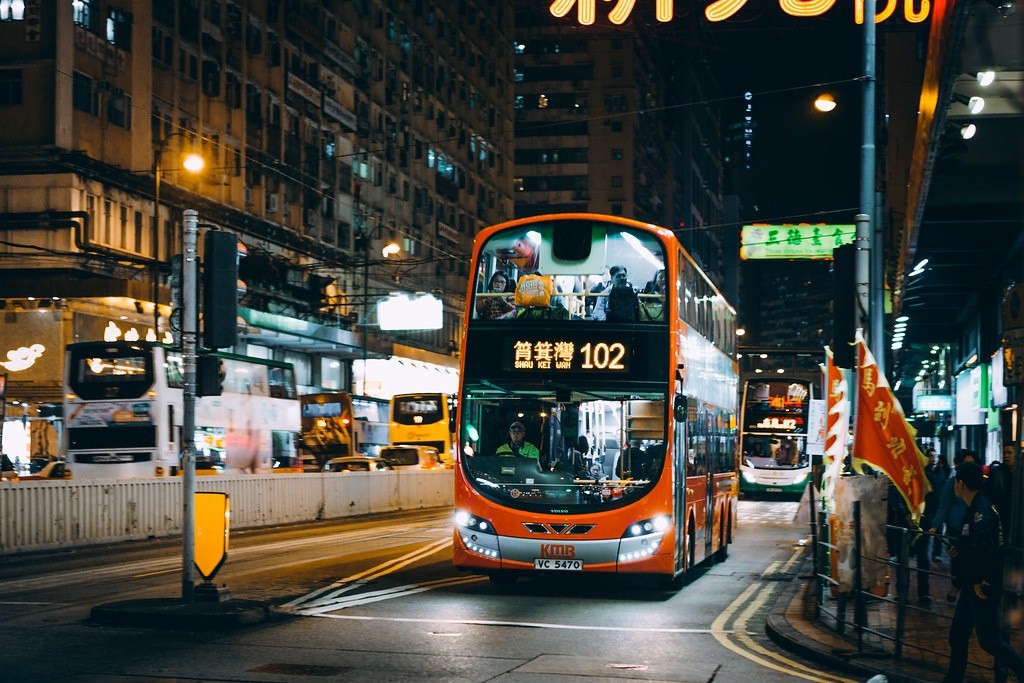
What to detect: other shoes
<box><xmin>947</xmin><ymin>585</ymin><xmax>960</xmax><ymax>602</ymax></box>
<box><xmin>920</xmin><ymin>595</ymin><xmax>933</xmax><ymax>604</ymax></box>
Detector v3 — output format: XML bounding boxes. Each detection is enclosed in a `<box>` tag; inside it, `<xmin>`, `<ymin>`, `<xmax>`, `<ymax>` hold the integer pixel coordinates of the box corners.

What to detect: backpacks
<box><xmin>605</xmin><ymin>281</ymin><xmax>638</xmax><ymax>323</ymax></box>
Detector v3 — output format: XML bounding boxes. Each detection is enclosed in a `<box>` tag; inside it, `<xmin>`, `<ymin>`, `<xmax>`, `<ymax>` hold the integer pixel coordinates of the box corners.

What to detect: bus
<box><xmin>60</xmin><ymin>339</ymin><xmax>305</xmax><ymax>473</ymax></box>
<box><xmin>739</xmin><ymin>368</ymin><xmax>826</xmax><ymax>495</ymax></box>
<box><xmin>298</xmin><ymin>391</ymin><xmax>391</xmax><ymax>463</ymax></box>
<box><xmin>453</xmin><ymin>216</ymin><xmax>744</xmax><ymax>582</ymax></box>
<box><xmin>390</xmin><ymin>392</ymin><xmax>459</xmax><ymax>469</ymax></box>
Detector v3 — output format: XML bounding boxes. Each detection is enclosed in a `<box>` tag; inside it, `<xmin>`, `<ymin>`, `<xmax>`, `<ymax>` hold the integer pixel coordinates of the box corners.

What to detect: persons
<box><xmin>495</xmin><ymin>423</ymin><xmax>542</xmax><ymax>470</ymax></box>
<box><xmin>572</xmin><ymin>431</ymin><xmax>606</xmax><ymax>478</ymax></box>
<box><xmin>478</xmin><ymin>268</ymin><xmax>666</xmax><ymax>320</ymax></box>
<box><xmin>615</xmin><ymin>429</ymin><xmax>657</xmax><ymax>479</ymax></box>
<box><xmin>887</xmin><ymin>444</ymin><xmax>1024</xmax><ymax>683</ymax></box>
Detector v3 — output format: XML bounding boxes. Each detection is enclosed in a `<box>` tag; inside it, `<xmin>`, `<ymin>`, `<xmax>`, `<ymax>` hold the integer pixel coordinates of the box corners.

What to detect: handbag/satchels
<box><xmin>638</xmin><ymin>303</ymin><xmax>665</xmax><ymax>321</ymax></box>
<box><xmin>524</xmin><ymin>302</ymin><xmax>569</xmax><ymax>320</ymax></box>
<box><xmin>514</xmin><ymin>274</ymin><xmax>551</xmax><ymax>306</ymax></box>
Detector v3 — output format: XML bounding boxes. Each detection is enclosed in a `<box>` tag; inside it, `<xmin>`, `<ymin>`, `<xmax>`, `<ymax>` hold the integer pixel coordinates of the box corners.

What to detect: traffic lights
<box><xmin>205</xmin><ymin>229</ymin><xmax>255</xmax><ymax>354</ymax></box>
<box><xmin>197</xmin><ymin>355</ymin><xmax>227</xmax><ymax>397</ymax></box>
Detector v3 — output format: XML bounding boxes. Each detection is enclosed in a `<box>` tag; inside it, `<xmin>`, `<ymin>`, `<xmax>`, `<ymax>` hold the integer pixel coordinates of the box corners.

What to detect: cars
<box><xmin>320</xmin><ymin>455</ymin><xmax>395</xmax><ymax>471</ymax></box>
<box><xmin>1</xmin><ymin>454</ymin><xmax>65</xmax><ymax>481</ymax></box>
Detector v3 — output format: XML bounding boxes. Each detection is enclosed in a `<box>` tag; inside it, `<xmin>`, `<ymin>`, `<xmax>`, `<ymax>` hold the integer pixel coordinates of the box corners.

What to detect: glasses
<box><xmin>494</xmin><ymin>279</ymin><xmax>506</xmax><ymax>285</ymax></box>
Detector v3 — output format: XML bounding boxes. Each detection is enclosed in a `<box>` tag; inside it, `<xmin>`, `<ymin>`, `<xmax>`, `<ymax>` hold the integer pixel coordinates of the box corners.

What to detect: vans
<box><xmin>378</xmin><ymin>445</ymin><xmax>452</xmax><ymax>470</ymax></box>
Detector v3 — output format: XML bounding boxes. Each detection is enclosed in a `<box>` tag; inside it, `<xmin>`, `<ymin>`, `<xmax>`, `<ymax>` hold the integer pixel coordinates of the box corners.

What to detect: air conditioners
<box><xmin>245</xmin><ymin>186</ymin><xmax>253</xmax><ymax>204</ymax></box>
<box><xmin>265</xmin><ymin>193</ymin><xmax>316</xmax><ymax>227</ymax></box>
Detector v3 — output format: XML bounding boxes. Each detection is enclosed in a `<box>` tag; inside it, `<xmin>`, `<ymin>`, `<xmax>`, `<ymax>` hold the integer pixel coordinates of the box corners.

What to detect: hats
<box><xmin>510</xmin><ymin>422</ymin><xmax>525</xmax><ymax>430</ymax></box>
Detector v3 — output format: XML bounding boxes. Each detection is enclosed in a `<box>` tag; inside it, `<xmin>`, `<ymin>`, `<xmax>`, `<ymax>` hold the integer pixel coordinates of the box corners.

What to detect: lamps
<box><xmin>946</xmin><ymin>118</ymin><xmax>976</xmax><ymax>140</ymax></box>
<box><xmin>950</xmin><ymin>92</ymin><xmax>986</xmax><ymax>114</ymax></box>
<box><xmin>985</xmin><ymin>0</ymin><xmax>1016</xmax><ymax>18</ymax></box>
<box><xmin>959</xmin><ymin>65</ymin><xmax>995</xmax><ymax>86</ymax></box>
<box><xmin>813</xmin><ymin>93</ymin><xmax>837</xmax><ymax>112</ymax></box>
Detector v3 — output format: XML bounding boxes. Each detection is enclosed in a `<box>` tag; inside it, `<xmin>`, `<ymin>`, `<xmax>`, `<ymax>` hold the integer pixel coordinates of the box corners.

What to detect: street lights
<box><xmin>814</xmin><ymin>74</ymin><xmax>886</xmax><ymax>393</ymax></box>
<box><xmin>360</xmin><ymin>225</ymin><xmax>403</xmax><ymax>392</ymax></box>
<box><xmin>153</xmin><ymin>132</ymin><xmax>211</xmax><ymax>341</ymax></box>
